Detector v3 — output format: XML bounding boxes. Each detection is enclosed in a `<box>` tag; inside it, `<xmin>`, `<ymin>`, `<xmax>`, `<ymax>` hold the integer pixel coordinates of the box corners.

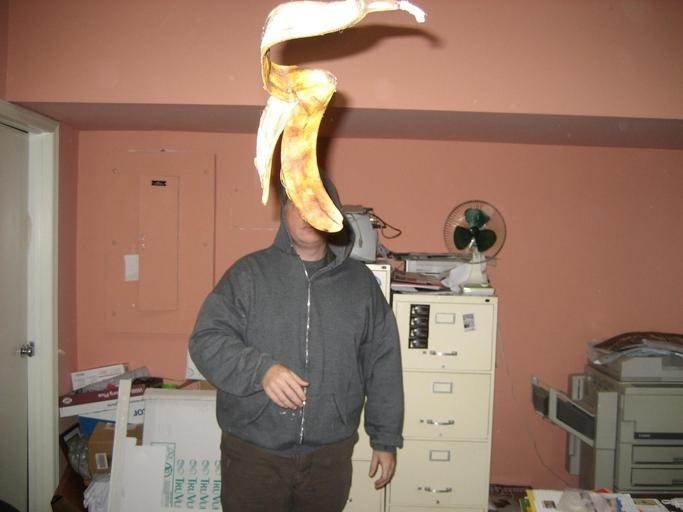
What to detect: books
<box><xmin>486</xmin><ymin>479</ymin><xmax>672</xmax><ymax>512</ymax></box>
<box><xmin>390</xmin><ymin>267</ymin><xmax>443</xmax><ymax>291</ymax></box>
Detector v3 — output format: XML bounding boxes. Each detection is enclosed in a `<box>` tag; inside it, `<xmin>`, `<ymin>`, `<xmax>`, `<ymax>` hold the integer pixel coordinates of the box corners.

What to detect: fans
<box><xmin>443</xmin><ymin>199</ymin><xmax>506</xmax><ymax>295</ymax></box>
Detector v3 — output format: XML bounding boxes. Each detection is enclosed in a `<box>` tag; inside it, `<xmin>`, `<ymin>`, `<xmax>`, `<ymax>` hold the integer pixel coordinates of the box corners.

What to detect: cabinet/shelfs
<box><xmin>337</xmin><ymin>262</ymin><xmax>393</xmax><ymax>512</ymax></box>
<box><xmin>385</xmin><ymin>294</ymin><xmax>498</xmax><ymax>511</ymax></box>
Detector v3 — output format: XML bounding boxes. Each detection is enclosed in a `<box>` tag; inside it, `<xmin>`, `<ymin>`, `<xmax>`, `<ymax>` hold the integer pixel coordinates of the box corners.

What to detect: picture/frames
<box><xmin>60</xmin><ymin>422</ymin><xmax>80</xmax><ymax>464</ymax></box>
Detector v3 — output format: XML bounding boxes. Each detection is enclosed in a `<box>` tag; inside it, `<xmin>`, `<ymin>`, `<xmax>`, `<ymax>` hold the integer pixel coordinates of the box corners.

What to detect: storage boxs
<box><xmin>86</xmin><ymin>420</ymin><xmax>142</xmax><ymax>477</ymax></box>
<box><xmin>50</xmin><ymin>470</ymin><xmax>90</xmax><ymax>512</ymax></box>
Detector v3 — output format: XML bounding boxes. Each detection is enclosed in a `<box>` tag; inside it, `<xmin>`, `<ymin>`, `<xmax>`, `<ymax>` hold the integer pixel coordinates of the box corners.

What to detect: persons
<box><xmin>185</xmin><ymin>177</ymin><xmax>403</xmax><ymax>512</ymax></box>
